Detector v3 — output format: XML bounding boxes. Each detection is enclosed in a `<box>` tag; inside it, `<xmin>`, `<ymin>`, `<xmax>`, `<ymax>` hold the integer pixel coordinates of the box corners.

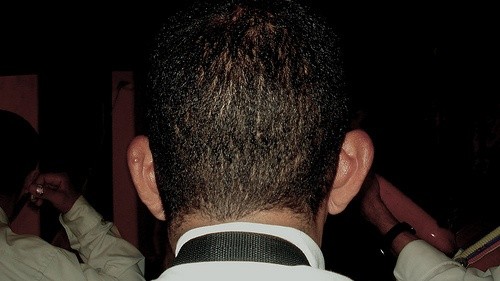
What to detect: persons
<box><xmin>126</xmin><ymin>0</ymin><xmax>374</xmax><ymax>281</ymax></box>
<box><xmin>0</xmin><ymin>109</ymin><xmax>146</xmax><ymax>281</ymax></box>
<box><xmin>355</xmin><ymin>175</ymin><xmax>500</xmax><ymax>281</ymax></box>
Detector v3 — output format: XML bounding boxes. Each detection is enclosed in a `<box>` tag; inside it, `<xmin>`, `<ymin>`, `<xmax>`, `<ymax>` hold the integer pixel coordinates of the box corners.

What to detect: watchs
<box><xmin>383</xmin><ymin>221</ymin><xmax>416</xmax><ymax>243</ymax></box>
